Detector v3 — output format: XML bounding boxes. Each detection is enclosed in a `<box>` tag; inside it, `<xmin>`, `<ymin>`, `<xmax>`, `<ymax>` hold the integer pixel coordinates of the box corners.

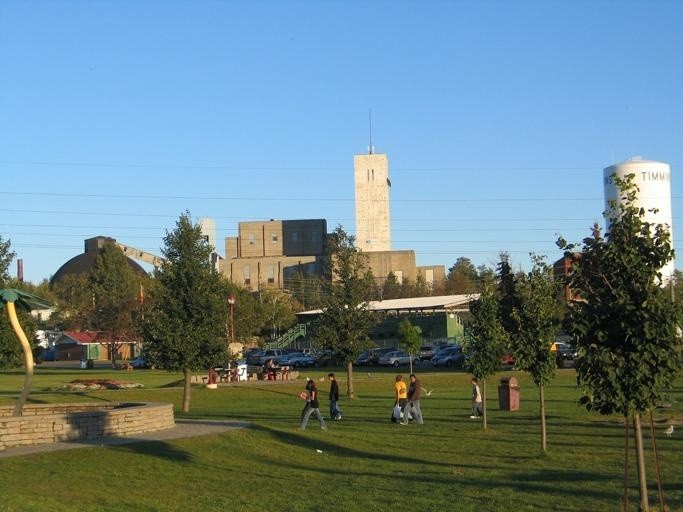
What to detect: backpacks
<box><xmin>312</xmin><ymin>400</ymin><xmax>319</xmax><ymax>408</ymax></box>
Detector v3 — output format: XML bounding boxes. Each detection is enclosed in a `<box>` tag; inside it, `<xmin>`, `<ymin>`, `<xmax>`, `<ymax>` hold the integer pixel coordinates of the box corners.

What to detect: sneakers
<box><xmin>334</xmin><ymin>415</ymin><xmax>341</xmax><ymax>421</ymax></box>
<box><xmin>296</xmin><ymin>426</ymin><xmax>306</xmax><ymax>432</ymax></box>
<box><xmin>399</xmin><ymin>421</ymin><xmax>408</xmax><ymax>426</ymax></box>
<box><xmin>321</xmin><ymin>425</ymin><xmax>327</xmax><ymax>430</ymax></box>
<box><xmin>470</xmin><ymin>415</ymin><xmax>482</xmax><ymax>419</ymax></box>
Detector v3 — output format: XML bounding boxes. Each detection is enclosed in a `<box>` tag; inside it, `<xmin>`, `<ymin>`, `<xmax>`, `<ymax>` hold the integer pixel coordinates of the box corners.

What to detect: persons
<box><xmin>392</xmin><ymin>375</ymin><xmax>423</xmax><ymax>425</ymax></box>
<box><xmin>329</xmin><ymin>374</ymin><xmax>342</xmax><ymax>421</ymax></box>
<box><xmin>296</xmin><ymin>380</ymin><xmax>327</xmax><ymax>432</ymax></box>
<box><xmin>469</xmin><ymin>378</ymin><xmax>483</xmax><ymax>418</ymax></box>
<box><xmin>266</xmin><ymin>359</ymin><xmax>274</xmax><ymax>369</ymax></box>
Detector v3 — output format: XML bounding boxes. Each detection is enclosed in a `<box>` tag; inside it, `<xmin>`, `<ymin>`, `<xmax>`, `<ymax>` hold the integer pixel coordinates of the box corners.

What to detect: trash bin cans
<box><xmin>498</xmin><ymin>377</ymin><xmax>520</xmax><ymax>411</ymax></box>
<box><xmin>80</xmin><ymin>359</ymin><xmax>94</xmax><ymax>369</ymax></box>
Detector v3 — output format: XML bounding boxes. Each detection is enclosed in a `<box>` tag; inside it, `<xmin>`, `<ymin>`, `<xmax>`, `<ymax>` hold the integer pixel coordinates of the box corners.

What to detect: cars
<box><xmin>242</xmin><ymin>343</ymin><xmax>466</xmax><ymax>369</ymax></box>
<box><xmin>556</xmin><ymin>343</ymin><xmax>578</xmax><ymax>361</ymax></box>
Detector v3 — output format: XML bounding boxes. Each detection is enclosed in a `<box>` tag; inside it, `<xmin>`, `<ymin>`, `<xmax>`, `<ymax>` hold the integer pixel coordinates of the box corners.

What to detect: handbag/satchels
<box><xmin>474</xmin><ymin>394</ymin><xmax>482</xmax><ymax>403</ymax></box>
<box><xmin>393</xmin><ymin>404</ymin><xmax>403</xmax><ymax>419</ymax></box>
